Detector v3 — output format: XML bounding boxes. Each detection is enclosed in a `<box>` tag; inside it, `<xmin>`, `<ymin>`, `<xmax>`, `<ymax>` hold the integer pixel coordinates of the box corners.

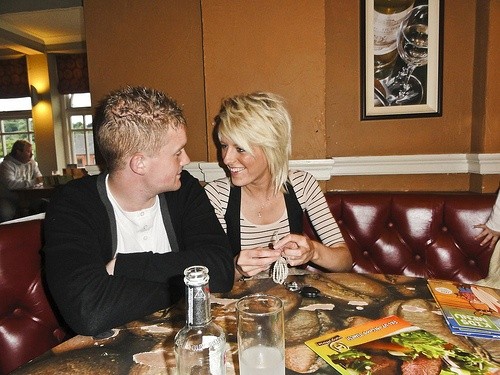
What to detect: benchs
<box><xmin>0</xmin><ymin>190</ymin><xmax>500</xmax><ymax>375</ymax></box>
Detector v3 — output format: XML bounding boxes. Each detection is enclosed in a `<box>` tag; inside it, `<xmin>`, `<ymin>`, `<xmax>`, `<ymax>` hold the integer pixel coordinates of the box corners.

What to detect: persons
<box><xmin>474</xmin><ymin>190</ymin><xmax>500</xmax><ymax>290</ymax></box>
<box><xmin>0</xmin><ymin>140</ymin><xmax>49</xmax><ymax>221</ymax></box>
<box><xmin>204</xmin><ymin>91</ymin><xmax>354</xmax><ymax>284</ymax></box>
<box><xmin>42</xmin><ymin>86</ymin><xmax>236</xmax><ymax>337</ymax></box>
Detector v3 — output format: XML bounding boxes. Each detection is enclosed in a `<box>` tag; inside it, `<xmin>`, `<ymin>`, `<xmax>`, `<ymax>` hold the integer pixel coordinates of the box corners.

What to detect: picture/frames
<box><xmin>360</xmin><ymin>0</ymin><xmax>443</xmax><ymax>121</ymax></box>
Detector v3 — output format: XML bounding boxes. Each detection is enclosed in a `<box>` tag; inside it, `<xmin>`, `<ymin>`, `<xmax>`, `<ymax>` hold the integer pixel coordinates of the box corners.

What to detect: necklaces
<box><xmin>246</xmin><ymin>189</ymin><xmax>271</xmax><ymax>218</ymax></box>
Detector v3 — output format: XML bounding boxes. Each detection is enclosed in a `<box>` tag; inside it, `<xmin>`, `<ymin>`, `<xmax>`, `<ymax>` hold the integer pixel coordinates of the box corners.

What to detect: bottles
<box><xmin>173</xmin><ymin>266</ymin><xmax>227</xmax><ymax>375</ymax></box>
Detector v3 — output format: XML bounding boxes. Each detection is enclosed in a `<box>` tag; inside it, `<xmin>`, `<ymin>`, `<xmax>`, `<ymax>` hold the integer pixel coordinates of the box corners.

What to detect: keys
<box><xmin>301</xmin><ymin>285</ymin><xmax>332</xmax><ymax>299</ymax></box>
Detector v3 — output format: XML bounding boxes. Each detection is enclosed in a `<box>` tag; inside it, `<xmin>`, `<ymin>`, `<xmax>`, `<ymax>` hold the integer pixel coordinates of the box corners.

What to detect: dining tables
<box><xmin>11</xmin><ymin>273</ymin><xmax>500</xmax><ymax>375</ymax></box>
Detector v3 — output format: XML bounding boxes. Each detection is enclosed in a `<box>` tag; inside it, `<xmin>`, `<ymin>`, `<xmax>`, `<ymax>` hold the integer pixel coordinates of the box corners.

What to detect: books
<box><xmin>303</xmin><ymin>280</ymin><xmax>500</xmax><ymax>375</ymax></box>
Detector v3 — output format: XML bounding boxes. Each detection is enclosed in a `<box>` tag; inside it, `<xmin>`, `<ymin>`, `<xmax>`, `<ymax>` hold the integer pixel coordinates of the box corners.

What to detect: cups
<box><xmin>234</xmin><ymin>293</ymin><xmax>285</xmax><ymax>375</ymax></box>
